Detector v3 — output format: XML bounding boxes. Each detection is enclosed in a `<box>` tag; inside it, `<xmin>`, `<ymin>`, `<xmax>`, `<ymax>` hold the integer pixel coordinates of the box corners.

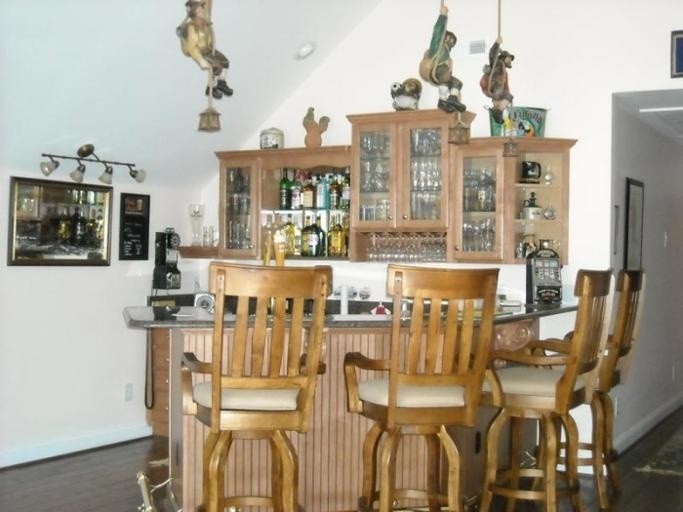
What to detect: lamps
<box><xmin>39</xmin><ymin>143</ymin><xmax>145</xmax><ymax>185</ymax></box>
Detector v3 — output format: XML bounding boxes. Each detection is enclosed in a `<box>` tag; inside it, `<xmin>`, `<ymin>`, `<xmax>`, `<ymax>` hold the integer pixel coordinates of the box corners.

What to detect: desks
<box><xmin>122</xmin><ymin>300</ymin><xmax>583</xmax><ymax>512</ymax></box>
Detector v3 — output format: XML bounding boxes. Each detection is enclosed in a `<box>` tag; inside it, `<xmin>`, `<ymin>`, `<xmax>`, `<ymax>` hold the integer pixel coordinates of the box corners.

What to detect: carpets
<box><xmin>636</xmin><ymin>422</ymin><xmax>682</xmax><ymax>476</ymax></box>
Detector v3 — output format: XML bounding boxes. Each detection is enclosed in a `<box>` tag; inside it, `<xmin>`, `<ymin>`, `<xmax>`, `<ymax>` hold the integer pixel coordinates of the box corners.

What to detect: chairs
<box><xmin>180</xmin><ymin>261</ymin><xmax>335</xmax><ymax>511</ymax></box>
<box><xmin>343</xmin><ymin>262</ymin><xmax>499</xmax><ymax>511</ymax></box>
<box><xmin>466</xmin><ymin>267</ymin><xmax>614</xmax><ymax>511</ymax></box>
<box><xmin>526</xmin><ymin>265</ymin><xmax>643</xmax><ymax>504</ymax></box>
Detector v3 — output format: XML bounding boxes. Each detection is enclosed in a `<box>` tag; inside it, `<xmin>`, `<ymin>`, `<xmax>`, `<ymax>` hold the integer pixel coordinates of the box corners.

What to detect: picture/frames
<box><xmin>671</xmin><ymin>29</ymin><xmax>683</xmax><ymax>78</ymax></box>
<box><xmin>6</xmin><ymin>176</ymin><xmax>113</xmax><ymax>268</ymax></box>
<box><xmin>626</xmin><ymin>176</ymin><xmax>644</xmax><ymax>276</ymax></box>
<box><xmin>118</xmin><ymin>190</ymin><xmax>151</xmax><ymax>262</ymax></box>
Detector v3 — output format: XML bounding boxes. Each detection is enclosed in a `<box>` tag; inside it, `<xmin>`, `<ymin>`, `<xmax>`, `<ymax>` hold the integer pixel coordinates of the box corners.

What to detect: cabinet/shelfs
<box><xmin>175</xmin><ymin>103</ymin><xmax>577</xmax><ymax>268</ymax></box>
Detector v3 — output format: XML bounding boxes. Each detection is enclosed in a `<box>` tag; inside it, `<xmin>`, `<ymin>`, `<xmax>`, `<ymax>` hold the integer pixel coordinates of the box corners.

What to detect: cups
<box><xmin>189</xmin><ymin>203</ymin><xmax>213</xmax><ymax>248</ymax></box>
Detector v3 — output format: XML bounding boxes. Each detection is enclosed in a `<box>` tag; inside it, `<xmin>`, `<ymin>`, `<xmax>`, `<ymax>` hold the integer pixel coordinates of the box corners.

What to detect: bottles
<box><xmin>227</xmin><ymin>168</ymin><xmax>250</xmax><ymax>250</ymax></box>
<box><xmin>58</xmin><ymin>201</ymin><xmax>104</xmax><ymax>248</ymax></box>
<box><xmin>262</xmin><ymin>167</ymin><xmax>349</xmax><ymax>258</ymax></box>
<box><xmin>464</xmin><ymin>168</ymin><xmax>495</xmax><ymax>212</ymax></box>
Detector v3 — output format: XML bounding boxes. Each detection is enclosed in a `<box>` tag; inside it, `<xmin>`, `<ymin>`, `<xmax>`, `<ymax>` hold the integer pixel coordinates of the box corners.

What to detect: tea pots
<box><xmin>518</xmin><ymin>161</ymin><xmax>541</xmax><ymax>184</ymax></box>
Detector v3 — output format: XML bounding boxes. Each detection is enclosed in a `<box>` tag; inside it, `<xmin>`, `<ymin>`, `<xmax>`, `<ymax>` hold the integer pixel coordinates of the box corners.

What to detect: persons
<box><xmin>182</xmin><ymin>0</ymin><xmax>233</xmax><ymax>98</ymax></box>
<box><xmin>420</xmin><ymin>5</ymin><xmax>466</xmax><ymax>114</ymax></box>
<box><xmin>480</xmin><ymin>37</ymin><xmax>514</xmax><ymax>125</ymax></box>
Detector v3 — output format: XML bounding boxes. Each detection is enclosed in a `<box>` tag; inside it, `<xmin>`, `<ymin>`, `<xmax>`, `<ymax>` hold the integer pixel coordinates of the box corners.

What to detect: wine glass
<box><xmin>360</xmin><ymin>232</ymin><xmax>447</xmax><ymax>262</ymax></box>
